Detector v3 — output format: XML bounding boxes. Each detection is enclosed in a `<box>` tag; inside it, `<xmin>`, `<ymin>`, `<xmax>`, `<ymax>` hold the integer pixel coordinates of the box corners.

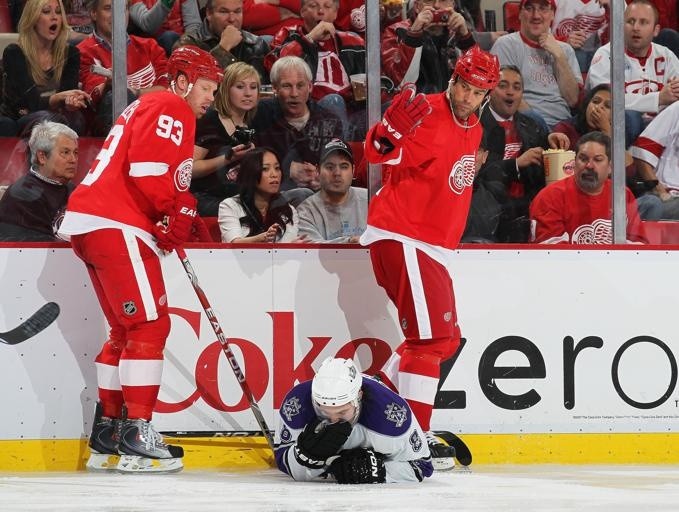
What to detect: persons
<box><xmin>217</xmin><ymin>145</ymin><xmax>300</xmax><ymax>246</ymax></box>
<box><xmin>56</xmin><ymin>43</ymin><xmax>224</xmax><ymax>474</ymax></box>
<box><xmin>188</xmin><ymin>59</ymin><xmax>261</xmax><ymax>216</ymax></box>
<box><xmin>274</xmin><ymin>356</ymin><xmax>433</xmax><ymax>486</ymax></box>
<box><xmin>294</xmin><ymin>136</ymin><xmax>373</xmax><ymax>244</ymax></box>
<box><xmin>460</xmin><ymin>128</ymin><xmax>505</xmax><ymax>245</ymax></box>
<box><xmin>0</xmin><ymin>0</ymin><xmax>366</xmax><ymax>142</ymax></box>
<box><xmin>0</xmin><ymin>120</ymin><xmax>83</xmax><ymax>243</ymax></box>
<box><xmin>249</xmin><ymin>55</ymin><xmax>347</xmax><ymax>205</ymax></box>
<box><xmin>380</xmin><ymin>0</ymin><xmax>679</xmax><ymax>222</ymax></box>
<box><xmin>359</xmin><ymin>44</ymin><xmax>502</xmax><ymax>473</ymax></box>
<box><xmin>528</xmin><ymin>132</ymin><xmax>650</xmax><ymax>250</ymax></box>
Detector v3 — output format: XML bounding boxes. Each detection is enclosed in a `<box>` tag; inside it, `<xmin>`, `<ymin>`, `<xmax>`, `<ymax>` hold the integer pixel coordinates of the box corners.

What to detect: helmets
<box><xmin>166</xmin><ymin>45</ymin><xmax>224</xmax><ymax>84</ymax></box>
<box><xmin>453</xmin><ymin>45</ymin><xmax>500</xmax><ymax>95</ymax></box>
<box><xmin>311</xmin><ymin>356</ymin><xmax>362</xmax><ymax>425</ymax></box>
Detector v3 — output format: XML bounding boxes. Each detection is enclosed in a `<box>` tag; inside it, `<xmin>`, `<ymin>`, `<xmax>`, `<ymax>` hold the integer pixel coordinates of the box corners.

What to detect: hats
<box><xmin>521</xmin><ymin>0</ymin><xmax>556</xmax><ymax>8</ymax></box>
<box><xmin>319</xmin><ymin>138</ymin><xmax>352</xmax><ymax>162</ymax></box>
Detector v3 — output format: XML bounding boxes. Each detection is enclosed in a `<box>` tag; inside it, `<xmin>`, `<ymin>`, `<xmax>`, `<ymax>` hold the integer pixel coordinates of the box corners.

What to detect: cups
<box><xmin>349</xmin><ymin>73</ymin><xmax>368</xmax><ymax>102</ymax></box>
<box><xmin>541</xmin><ymin>152</ymin><xmax>577</xmax><ymax>187</ymax></box>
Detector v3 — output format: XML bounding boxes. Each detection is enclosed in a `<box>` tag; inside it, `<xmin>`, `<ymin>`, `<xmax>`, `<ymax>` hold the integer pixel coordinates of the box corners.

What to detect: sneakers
<box><xmin>88</xmin><ymin>401</ymin><xmax>121</xmax><ymax>455</ymax></box>
<box><xmin>424</xmin><ymin>430</ymin><xmax>455</xmax><ymax>458</ymax></box>
<box><xmin>117</xmin><ymin>405</ymin><xmax>183</xmax><ymax>458</ymax></box>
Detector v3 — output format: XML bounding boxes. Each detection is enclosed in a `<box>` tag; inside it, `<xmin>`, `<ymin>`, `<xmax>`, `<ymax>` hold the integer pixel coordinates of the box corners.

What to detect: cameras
<box><xmin>432</xmin><ymin>9</ymin><xmax>451</xmax><ymax>23</ymax></box>
<box><xmin>234</xmin><ymin>125</ymin><xmax>256</xmax><ymax>144</ymax></box>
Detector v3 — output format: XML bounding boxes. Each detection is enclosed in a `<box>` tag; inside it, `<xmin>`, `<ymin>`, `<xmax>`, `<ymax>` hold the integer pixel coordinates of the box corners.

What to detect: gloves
<box><xmin>374</xmin><ymin>82</ymin><xmax>432</xmax><ymax>153</ymax></box>
<box><xmin>293</xmin><ymin>418</ymin><xmax>352</xmax><ymax>469</ymax></box>
<box><xmin>325</xmin><ymin>447</ymin><xmax>386</xmax><ymax>483</ymax></box>
<box><xmin>155</xmin><ymin>197</ymin><xmax>197</xmax><ymax>251</ymax></box>
